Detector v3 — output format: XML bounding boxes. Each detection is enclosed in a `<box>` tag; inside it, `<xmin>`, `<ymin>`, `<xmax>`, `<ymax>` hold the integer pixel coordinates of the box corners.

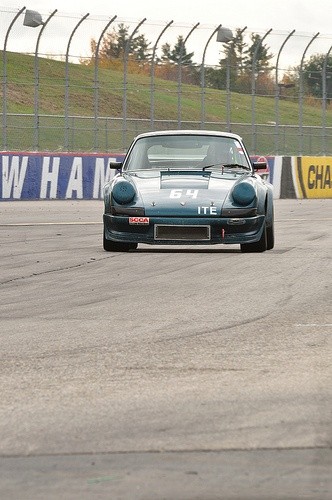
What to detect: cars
<box><xmin>102</xmin><ymin>129</ymin><xmax>278</xmax><ymax>252</ymax></box>
